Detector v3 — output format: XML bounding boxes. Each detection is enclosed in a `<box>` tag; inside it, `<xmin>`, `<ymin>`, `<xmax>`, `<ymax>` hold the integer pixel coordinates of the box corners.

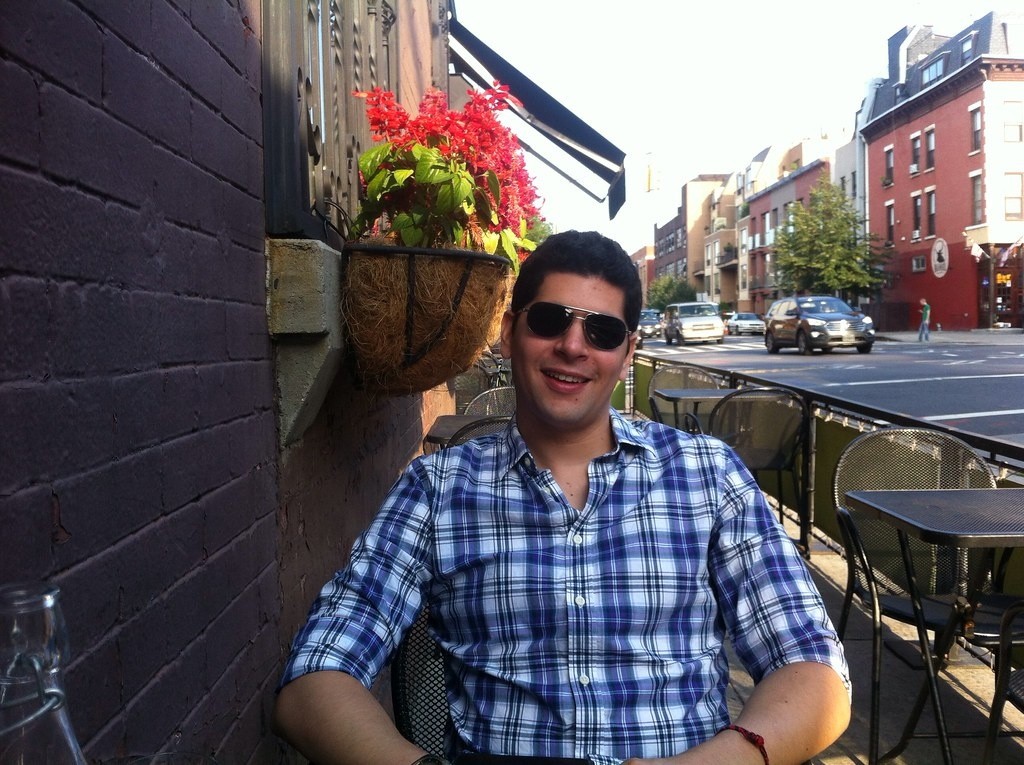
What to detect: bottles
<box><xmin>0</xmin><ymin>582</ymin><xmax>86</xmax><ymax>765</ymax></box>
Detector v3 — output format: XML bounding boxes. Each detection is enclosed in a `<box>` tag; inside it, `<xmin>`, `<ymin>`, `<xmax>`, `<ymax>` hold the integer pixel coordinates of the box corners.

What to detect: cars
<box><xmin>635</xmin><ymin>302</ymin><xmax>766</xmax><ymax>349</ymax></box>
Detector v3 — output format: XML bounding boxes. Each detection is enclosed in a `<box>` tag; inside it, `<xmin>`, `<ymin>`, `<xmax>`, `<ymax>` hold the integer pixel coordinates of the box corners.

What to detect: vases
<box><xmin>351</xmin><ymin>242</ymin><xmax>507</xmax><ymax>397</ymax></box>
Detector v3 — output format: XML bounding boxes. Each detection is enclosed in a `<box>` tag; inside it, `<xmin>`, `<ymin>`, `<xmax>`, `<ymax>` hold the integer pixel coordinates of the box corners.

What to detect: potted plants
<box><xmin>880</xmin><ymin>177</ymin><xmax>892</xmax><ymax>185</ymax></box>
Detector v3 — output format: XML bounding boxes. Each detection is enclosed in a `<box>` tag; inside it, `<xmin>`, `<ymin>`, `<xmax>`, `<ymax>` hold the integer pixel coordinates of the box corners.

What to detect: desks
<box><xmin>843</xmin><ymin>488</ymin><xmax>1024</xmax><ymax>765</ymax></box>
<box><xmin>426</xmin><ymin>415</ymin><xmax>509</xmax><ymax>445</ymax></box>
<box><xmin>654</xmin><ymin>387</ymin><xmax>800</xmax><ymax>524</ymax></box>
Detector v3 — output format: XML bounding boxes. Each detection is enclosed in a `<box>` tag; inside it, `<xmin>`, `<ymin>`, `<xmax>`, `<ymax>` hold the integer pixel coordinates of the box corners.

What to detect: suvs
<box><xmin>764</xmin><ymin>294</ymin><xmax>875</xmax><ymax>357</ymax></box>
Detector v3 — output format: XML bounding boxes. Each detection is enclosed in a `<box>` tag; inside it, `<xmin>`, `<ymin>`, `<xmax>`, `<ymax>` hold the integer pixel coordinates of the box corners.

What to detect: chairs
<box><xmin>463</xmin><ymin>385</ymin><xmax>516</xmax><ymax>418</ymax></box>
<box><xmin>977</xmin><ymin>602</ymin><xmax>1024</xmax><ymax>765</ymax></box>
<box><xmin>646</xmin><ymin>365</ymin><xmax>720</xmax><ymax>437</ymax></box>
<box><xmin>446</xmin><ymin>416</ymin><xmax>512</xmax><ymax>447</ymax></box>
<box><xmin>830</xmin><ymin>427</ymin><xmax>1014</xmax><ymax>765</ymax></box>
<box><xmin>684</xmin><ymin>386</ymin><xmax>811</xmax><ymax>560</ymax></box>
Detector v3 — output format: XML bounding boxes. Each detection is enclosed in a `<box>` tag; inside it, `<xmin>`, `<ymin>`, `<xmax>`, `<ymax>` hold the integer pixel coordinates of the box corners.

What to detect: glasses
<box><xmin>516</xmin><ymin>301</ymin><xmax>633</xmax><ymax>350</ymax></box>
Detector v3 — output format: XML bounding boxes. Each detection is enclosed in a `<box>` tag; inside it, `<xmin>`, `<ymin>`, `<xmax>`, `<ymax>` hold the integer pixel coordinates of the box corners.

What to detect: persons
<box><xmin>268</xmin><ymin>229</ymin><xmax>853</xmax><ymax>765</ymax></box>
<box><xmin>918</xmin><ymin>298</ymin><xmax>931</xmax><ymax>342</ymax></box>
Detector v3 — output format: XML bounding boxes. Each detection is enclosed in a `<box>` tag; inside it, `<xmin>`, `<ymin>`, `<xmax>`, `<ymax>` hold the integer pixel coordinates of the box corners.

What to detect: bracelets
<box><xmin>715</xmin><ymin>725</ymin><xmax>769</xmax><ymax>765</ymax></box>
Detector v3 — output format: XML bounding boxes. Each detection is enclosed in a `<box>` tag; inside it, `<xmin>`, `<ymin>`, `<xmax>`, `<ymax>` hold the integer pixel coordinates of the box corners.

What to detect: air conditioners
<box><xmin>912</xmin><ymin>231</ymin><xmax>920</xmax><ymax>239</ymax></box>
<box><xmin>909</xmin><ymin>164</ymin><xmax>920</xmax><ymax>174</ymax></box>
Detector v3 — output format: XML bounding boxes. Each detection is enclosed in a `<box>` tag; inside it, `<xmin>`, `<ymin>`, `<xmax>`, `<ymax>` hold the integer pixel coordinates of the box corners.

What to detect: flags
<box><xmin>971</xmin><ymin>242</ymin><xmax>983</xmax><ymax>263</ymax></box>
<box><xmin>1000</xmin><ymin>239</ymin><xmax>1022</xmax><ymax>267</ymax></box>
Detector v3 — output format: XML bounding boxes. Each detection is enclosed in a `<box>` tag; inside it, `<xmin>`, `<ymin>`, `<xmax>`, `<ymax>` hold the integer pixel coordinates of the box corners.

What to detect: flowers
<box><xmin>348</xmin><ymin>78</ymin><xmax>542</xmax><ymax>271</ymax></box>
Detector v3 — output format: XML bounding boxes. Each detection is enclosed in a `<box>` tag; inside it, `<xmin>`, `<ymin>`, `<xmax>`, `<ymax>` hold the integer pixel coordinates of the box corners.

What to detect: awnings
<box><xmin>448</xmin><ymin>12</ymin><xmax>627</xmax><ymax>220</ymax></box>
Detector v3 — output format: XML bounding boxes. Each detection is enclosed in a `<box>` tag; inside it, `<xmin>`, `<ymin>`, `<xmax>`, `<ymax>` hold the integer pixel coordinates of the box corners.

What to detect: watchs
<box><xmin>411</xmin><ymin>755</ymin><xmax>453</xmax><ymax>765</ymax></box>
